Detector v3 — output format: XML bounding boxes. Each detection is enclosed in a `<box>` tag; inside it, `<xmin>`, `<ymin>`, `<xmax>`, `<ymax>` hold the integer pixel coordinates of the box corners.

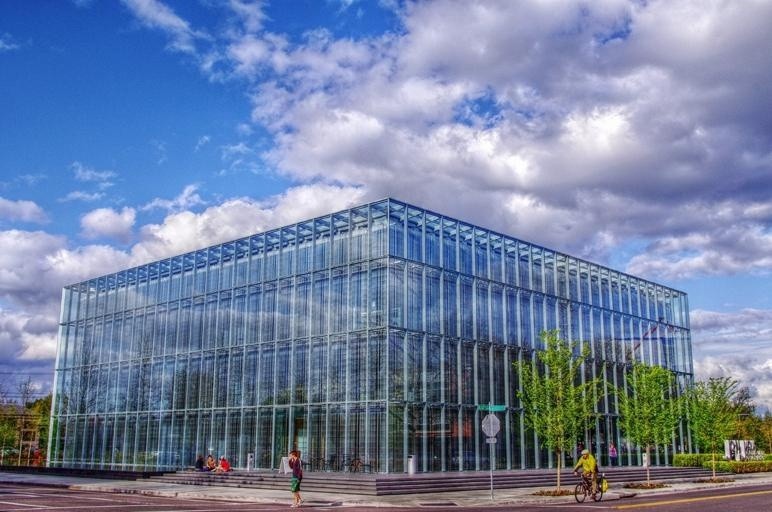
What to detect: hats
<box><xmin>581</xmin><ymin>450</ymin><xmax>589</xmax><ymax>454</ymax></box>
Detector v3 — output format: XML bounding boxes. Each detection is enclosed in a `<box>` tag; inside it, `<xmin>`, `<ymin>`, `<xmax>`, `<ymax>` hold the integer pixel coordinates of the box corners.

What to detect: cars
<box><xmin>0</xmin><ymin>446</ymin><xmax>20</xmax><ymax>458</ymax></box>
<box><xmin>17</xmin><ymin>446</ymin><xmax>46</xmax><ymax>463</ymax></box>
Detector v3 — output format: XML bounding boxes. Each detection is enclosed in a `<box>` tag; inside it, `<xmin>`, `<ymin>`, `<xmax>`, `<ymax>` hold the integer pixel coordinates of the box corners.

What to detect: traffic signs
<box><xmin>476</xmin><ymin>403</ymin><xmax>490</xmax><ymax>411</ymax></box>
<box><xmin>490</xmin><ymin>404</ymin><xmax>507</xmax><ymax>412</ymax></box>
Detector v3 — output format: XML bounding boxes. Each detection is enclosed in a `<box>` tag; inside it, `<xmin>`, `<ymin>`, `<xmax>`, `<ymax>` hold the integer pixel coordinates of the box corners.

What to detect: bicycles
<box><xmin>574</xmin><ymin>470</ymin><xmax>604</xmax><ymax>502</ymax></box>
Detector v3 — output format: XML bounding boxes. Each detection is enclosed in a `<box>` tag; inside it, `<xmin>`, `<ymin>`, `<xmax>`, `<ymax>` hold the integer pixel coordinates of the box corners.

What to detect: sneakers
<box><xmin>291</xmin><ymin>500</ymin><xmax>304</xmax><ymax>507</ymax></box>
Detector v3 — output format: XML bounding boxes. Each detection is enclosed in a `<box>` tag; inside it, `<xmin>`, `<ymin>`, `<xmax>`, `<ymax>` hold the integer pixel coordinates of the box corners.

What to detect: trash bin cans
<box><xmin>408</xmin><ymin>455</ymin><xmax>416</xmax><ymax>474</ymax></box>
<box><xmin>643</xmin><ymin>454</ymin><xmax>647</xmax><ymax>467</ymax></box>
<box><xmin>248</xmin><ymin>453</ymin><xmax>254</xmax><ymax>470</ymax></box>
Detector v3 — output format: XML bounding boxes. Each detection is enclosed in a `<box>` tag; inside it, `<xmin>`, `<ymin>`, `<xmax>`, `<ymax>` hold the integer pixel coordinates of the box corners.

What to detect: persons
<box><xmin>570</xmin><ymin>448</ymin><xmax>599</xmax><ymax>498</ymax></box>
<box><xmin>215</xmin><ymin>456</ymin><xmax>231</xmax><ymax>475</ymax></box>
<box><xmin>609</xmin><ymin>442</ymin><xmax>617</xmax><ymax>466</ymax></box>
<box><xmin>288</xmin><ymin>450</ymin><xmax>305</xmax><ymax>508</ymax></box>
<box><xmin>195</xmin><ymin>455</ymin><xmax>204</xmax><ymax>471</ymax></box>
<box><xmin>200</xmin><ymin>455</ymin><xmax>216</xmax><ymax>472</ymax></box>
